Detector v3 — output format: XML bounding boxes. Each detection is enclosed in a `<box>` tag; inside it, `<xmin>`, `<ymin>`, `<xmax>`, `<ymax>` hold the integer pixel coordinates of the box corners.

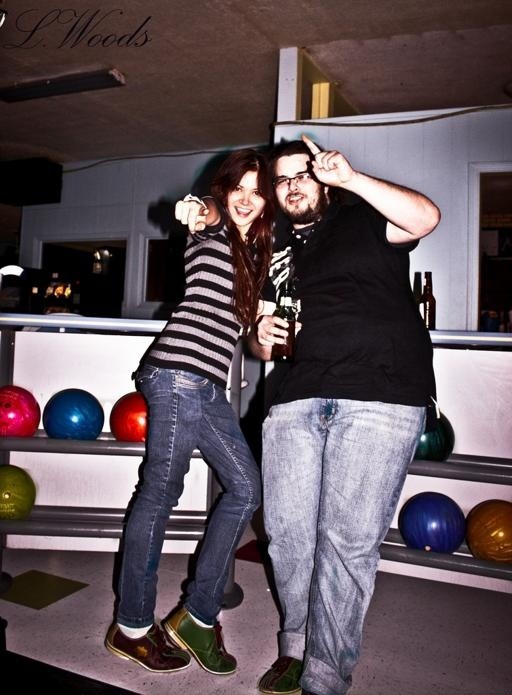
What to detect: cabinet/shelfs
<box><xmin>0</xmin><ymin>306</ymin><xmax>243</xmax><ymax>610</ymax></box>
<box><xmin>265</xmin><ymin>331</ymin><xmax>512</xmax><ymax>595</ymax></box>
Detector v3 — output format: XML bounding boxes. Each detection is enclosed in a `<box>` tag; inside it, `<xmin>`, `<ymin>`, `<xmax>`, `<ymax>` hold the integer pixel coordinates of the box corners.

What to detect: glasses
<box><xmin>272</xmin><ymin>173</ymin><xmax>313</xmax><ymax>188</ymax></box>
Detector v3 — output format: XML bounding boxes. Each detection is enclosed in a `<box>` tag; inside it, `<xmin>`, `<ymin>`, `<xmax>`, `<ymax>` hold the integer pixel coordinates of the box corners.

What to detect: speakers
<box><xmin>0</xmin><ymin>157</ymin><xmax>63</xmax><ymax>207</ymax></box>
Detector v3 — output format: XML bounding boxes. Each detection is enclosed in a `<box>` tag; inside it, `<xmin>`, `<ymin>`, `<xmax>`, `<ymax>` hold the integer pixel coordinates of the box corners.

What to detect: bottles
<box><xmin>269</xmin><ymin>280</ymin><xmax>296</xmax><ymax>364</ymax></box>
<box><xmin>412</xmin><ymin>272</ymin><xmax>426</xmax><ymax>330</ymax></box>
<box><xmin>422</xmin><ymin>272</ymin><xmax>436</xmax><ymax>330</ymax></box>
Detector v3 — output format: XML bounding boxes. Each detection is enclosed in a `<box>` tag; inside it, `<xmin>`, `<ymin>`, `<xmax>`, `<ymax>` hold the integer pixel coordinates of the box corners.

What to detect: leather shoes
<box><xmin>104</xmin><ymin>607</ymin><xmax>237</xmax><ymax>675</ymax></box>
<box><xmin>258</xmin><ymin>655</ymin><xmax>303</xmax><ymax>694</ymax></box>
